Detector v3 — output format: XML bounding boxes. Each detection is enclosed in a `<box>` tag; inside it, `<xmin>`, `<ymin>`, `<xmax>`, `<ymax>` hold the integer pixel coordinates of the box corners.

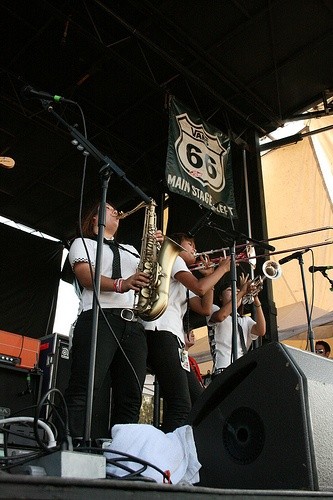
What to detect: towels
<box><xmin>100</xmin><ymin>423</ymin><xmax>203</xmax><ymax>486</ymax></box>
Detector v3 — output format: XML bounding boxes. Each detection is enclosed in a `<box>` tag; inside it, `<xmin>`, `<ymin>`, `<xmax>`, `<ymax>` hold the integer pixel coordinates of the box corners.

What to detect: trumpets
<box><xmin>240</xmin><ymin>260</ymin><xmax>281</xmax><ymax>305</ymax></box>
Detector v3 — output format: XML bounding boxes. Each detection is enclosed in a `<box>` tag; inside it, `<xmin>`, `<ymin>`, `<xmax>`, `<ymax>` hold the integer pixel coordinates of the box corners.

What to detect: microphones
<box><xmin>186</xmin><ymin>202</ymin><xmax>219</xmax><ymax>239</ymax></box>
<box><xmin>278</xmin><ymin>248</ymin><xmax>310</xmax><ymax>265</ymax></box>
<box><xmin>309</xmin><ymin>266</ymin><xmax>333</xmax><ymax>273</ymax></box>
<box><xmin>20</xmin><ymin>85</ymin><xmax>72</xmax><ymax>103</ymax></box>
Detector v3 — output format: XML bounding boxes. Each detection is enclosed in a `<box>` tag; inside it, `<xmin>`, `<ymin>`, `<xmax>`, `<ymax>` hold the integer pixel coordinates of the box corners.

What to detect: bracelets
<box><xmin>254</xmin><ymin>303</ymin><xmax>261</xmax><ymax>309</ymax></box>
<box><xmin>114</xmin><ymin>278</ymin><xmax>125</xmax><ymax>293</ymax></box>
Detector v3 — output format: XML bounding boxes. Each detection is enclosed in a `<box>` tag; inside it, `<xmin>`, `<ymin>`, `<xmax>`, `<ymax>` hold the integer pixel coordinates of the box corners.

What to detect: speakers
<box><xmin>182</xmin><ymin>340</ymin><xmax>333</xmax><ymax>491</ymax></box>
<box><xmin>0</xmin><ymin>369</ymin><xmax>37</xmax><ymax>417</ymax></box>
<box><xmin>52</xmin><ymin>362</ymin><xmax>111</xmax><ymax>438</ymax></box>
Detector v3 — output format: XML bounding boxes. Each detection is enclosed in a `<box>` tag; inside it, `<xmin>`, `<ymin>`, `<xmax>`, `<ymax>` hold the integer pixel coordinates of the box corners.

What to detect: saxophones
<box><xmin>116</xmin><ymin>197</ymin><xmax>189</xmax><ymax>322</ymax></box>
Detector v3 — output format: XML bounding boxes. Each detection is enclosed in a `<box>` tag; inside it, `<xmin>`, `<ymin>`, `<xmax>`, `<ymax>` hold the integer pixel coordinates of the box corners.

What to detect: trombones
<box><xmin>188</xmin><ymin>225</ymin><xmax>333</xmax><ymax>271</ymax></box>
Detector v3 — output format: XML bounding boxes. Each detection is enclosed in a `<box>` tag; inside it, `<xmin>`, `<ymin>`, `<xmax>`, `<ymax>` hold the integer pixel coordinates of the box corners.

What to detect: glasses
<box><xmin>315</xmin><ymin>349</ymin><xmax>324</xmax><ymax>353</ymax></box>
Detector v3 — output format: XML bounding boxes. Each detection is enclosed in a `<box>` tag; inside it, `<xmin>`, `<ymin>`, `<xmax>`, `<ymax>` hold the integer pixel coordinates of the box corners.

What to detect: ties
<box><xmin>103</xmin><ymin>238</ymin><xmax>122</xmax><ymax>279</ymax></box>
<box><xmin>238</xmin><ymin>322</ymin><xmax>248</xmax><ymax>356</ymax></box>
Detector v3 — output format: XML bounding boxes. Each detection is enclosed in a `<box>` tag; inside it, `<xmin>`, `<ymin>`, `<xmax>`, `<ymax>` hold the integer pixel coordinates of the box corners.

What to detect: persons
<box><xmin>67</xmin><ymin>203</ymin><xmax>165</xmax><ymax>452</ymax></box>
<box><xmin>142</xmin><ymin>232</ymin><xmax>240</xmax><ymax>433</ymax></box>
<box><xmin>206</xmin><ymin>271</ymin><xmax>267</xmax><ymax>378</ymax></box>
<box><xmin>315</xmin><ymin>339</ymin><xmax>331</xmax><ymax>359</ymax></box>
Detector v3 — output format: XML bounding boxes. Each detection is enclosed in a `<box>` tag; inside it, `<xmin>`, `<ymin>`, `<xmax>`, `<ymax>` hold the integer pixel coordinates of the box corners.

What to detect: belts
<box><xmin>101</xmin><ymin>307</ymin><xmax>146</xmax><ymax>328</ymax></box>
